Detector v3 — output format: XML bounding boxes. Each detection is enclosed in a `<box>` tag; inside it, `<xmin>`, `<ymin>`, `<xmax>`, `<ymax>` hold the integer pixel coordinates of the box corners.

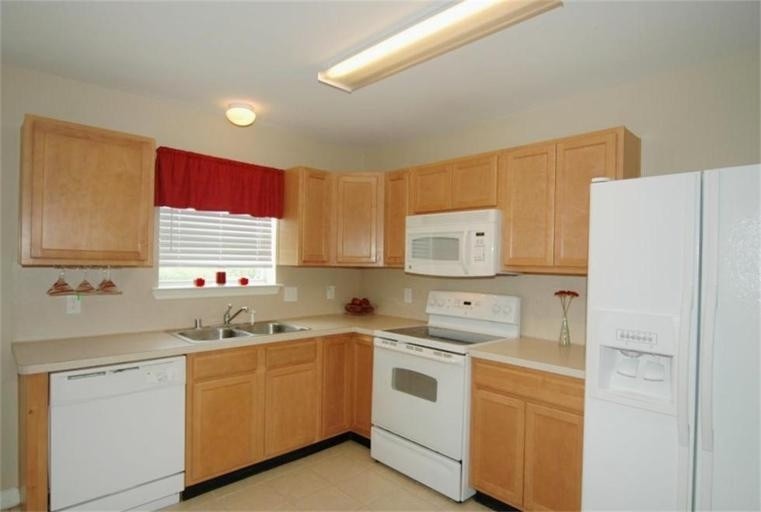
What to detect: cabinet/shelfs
<box><xmin>385</xmin><ymin>170</ymin><xmax>410</xmax><ymax>268</ymax></box>
<box><xmin>20</xmin><ymin>113</ymin><xmax>155</xmax><ymax>270</ymax></box>
<box><xmin>353</xmin><ymin>337</ymin><xmax>372</xmax><ymax>439</ymax></box>
<box><xmin>277</xmin><ymin>164</ymin><xmax>329</xmax><ymax>267</ymax></box>
<box><xmin>188</xmin><ymin>339</ymin><xmax>321</xmax><ymax>486</ymax></box>
<box><xmin>505</xmin><ymin>128</ymin><xmax>648</xmax><ymax>279</ymax></box>
<box><xmin>321</xmin><ymin>330</ymin><xmax>353</xmax><ymax>439</ymax></box>
<box><xmin>473</xmin><ymin>355</ymin><xmax>583</xmax><ymax>512</ymax></box>
<box><xmin>327</xmin><ymin>171</ymin><xmax>389</xmax><ymax>267</ymax></box>
<box><xmin>410</xmin><ymin>152</ymin><xmax>501</xmax><ymax>214</ymax></box>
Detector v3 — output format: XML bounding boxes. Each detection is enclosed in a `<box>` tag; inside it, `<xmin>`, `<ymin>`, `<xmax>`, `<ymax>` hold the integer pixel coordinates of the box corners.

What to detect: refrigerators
<box><xmin>580</xmin><ymin>158</ymin><xmax>760</xmax><ymax>512</ymax></box>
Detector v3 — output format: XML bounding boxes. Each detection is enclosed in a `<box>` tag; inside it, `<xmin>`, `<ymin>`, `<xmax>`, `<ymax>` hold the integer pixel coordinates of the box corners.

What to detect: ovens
<box><xmin>371</xmin><ymin>336</ymin><xmax>466</xmax><ymax>461</ymax></box>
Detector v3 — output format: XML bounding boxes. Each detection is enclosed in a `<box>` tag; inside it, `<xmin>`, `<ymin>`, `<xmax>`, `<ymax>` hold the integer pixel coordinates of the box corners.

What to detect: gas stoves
<box><xmin>373</xmin><ymin>314</ymin><xmax>523</xmax><ymax>354</ymax></box>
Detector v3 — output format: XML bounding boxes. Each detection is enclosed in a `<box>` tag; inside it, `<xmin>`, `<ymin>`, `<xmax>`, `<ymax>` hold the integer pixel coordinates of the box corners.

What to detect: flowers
<box><xmin>555</xmin><ymin>290</ymin><xmax>579</xmax><ymax>317</ymax></box>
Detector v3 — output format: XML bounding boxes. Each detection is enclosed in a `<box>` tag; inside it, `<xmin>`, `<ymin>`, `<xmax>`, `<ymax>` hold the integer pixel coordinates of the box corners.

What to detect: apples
<box><xmin>345</xmin><ymin>298</ymin><xmax>373</xmax><ymax>313</ymax></box>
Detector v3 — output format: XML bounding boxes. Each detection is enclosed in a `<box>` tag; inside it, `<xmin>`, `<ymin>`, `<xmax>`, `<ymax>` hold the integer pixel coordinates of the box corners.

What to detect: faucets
<box><xmin>223</xmin><ymin>302</ymin><xmax>249</xmax><ymax>326</ymax></box>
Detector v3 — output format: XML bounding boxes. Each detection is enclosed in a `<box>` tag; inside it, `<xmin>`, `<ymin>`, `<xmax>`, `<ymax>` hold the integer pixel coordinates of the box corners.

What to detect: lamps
<box><xmin>315</xmin><ymin>2</ymin><xmax>565</xmax><ymax>95</ymax></box>
<box><xmin>223</xmin><ymin>100</ymin><xmax>259</xmax><ymax>126</ymax></box>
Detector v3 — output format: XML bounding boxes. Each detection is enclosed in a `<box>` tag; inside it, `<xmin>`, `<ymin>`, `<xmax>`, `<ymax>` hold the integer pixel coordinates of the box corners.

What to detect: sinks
<box><xmin>235</xmin><ymin>320</ymin><xmax>311</xmax><ymax>335</ymax></box>
<box><xmin>173</xmin><ymin>327</ymin><xmax>250</xmax><ymax>344</ymax></box>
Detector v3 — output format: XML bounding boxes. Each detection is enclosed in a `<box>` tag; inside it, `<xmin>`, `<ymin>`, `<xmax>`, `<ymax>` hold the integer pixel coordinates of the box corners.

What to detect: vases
<box><xmin>559</xmin><ymin>314</ymin><xmax>570</xmax><ymax>345</ymax></box>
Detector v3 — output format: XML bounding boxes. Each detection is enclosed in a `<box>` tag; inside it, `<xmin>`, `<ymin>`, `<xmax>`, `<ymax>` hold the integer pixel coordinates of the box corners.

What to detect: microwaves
<box><xmin>403</xmin><ymin>208</ymin><xmax>516</xmax><ymax>279</ymax></box>
